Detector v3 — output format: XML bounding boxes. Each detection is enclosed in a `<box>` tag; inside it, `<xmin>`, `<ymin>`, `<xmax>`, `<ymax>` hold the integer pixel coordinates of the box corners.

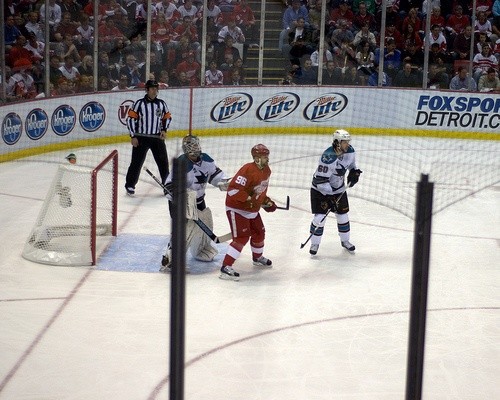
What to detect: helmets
<box><xmin>183</xmin><ymin>135</ymin><xmax>201</xmax><ymax>153</ymax></box>
<box><xmin>333</xmin><ymin>129</ymin><xmax>352</xmax><ymax>143</ymax></box>
<box><xmin>251</xmin><ymin>144</ymin><xmax>271</xmax><ymax>157</ymax></box>
<box><xmin>146</xmin><ymin>79</ymin><xmax>159</xmax><ymax>88</ymax></box>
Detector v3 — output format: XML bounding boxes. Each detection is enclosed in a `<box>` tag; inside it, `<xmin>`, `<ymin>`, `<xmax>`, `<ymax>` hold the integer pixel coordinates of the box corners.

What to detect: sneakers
<box><xmin>341</xmin><ymin>241</ymin><xmax>356</xmax><ymax>254</ymax></box>
<box><xmin>125</xmin><ymin>185</ymin><xmax>136</xmax><ymax>197</ymax></box>
<box><xmin>309</xmin><ymin>244</ymin><xmax>319</xmax><ymax>256</ymax></box>
<box><xmin>252</xmin><ymin>256</ymin><xmax>273</xmax><ymax>268</ymax></box>
<box><xmin>218</xmin><ymin>266</ymin><xmax>241</xmax><ymax>281</ymax></box>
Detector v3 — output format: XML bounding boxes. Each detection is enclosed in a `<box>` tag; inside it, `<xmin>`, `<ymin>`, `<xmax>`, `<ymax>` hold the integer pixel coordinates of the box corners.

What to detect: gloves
<box><xmin>347</xmin><ymin>169</ymin><xmax>363</xmax><ymax>188</ymax></box>
<box><xmin>263</xmin><ymin>197</ymin><xmax>278</xmax><ymax>213</ymax></box>
<box><xmin>326</xmin><ymin>196</ymin><xmax>339</xmax><ymax>212</ymax></box>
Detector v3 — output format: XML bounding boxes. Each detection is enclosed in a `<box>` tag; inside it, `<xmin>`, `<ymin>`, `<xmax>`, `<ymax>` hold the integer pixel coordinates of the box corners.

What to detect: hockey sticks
<box><xmin>260</xmin><ymin>196</ymin><xmax>290</xmax><ymax>210</ymax></box>
<box><xmin>300</xmin><ymin>181</ymin><xmax>351</xmax><ymax>248</ymax></box>
<box><xmin>143</xmin><ymin>165</ymin><xmax>233</xmax><ymax>244</ymax></box>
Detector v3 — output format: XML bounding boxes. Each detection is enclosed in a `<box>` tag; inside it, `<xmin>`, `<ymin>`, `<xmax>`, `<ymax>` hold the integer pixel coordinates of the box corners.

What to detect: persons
<box><xmin>0</xmin><ymin>0</ymin><xmax>500</xmax><ymax>108</ymax></box>
<box><xmin>155</xmin><ymin>134</ymin><xmax>233</xmax><ymax>270</ymax></box>
<box><xmin>123</xmin><ymin>78</ymin><xmax>170</xmax><ymax>197</ymax></box>
<box><xmin>308</xmin><ymin>128</ymin><xmax>360</xmax><ymax>256</ymax></box>
<box><xmin>219</xmin><ymin>143</ymin><xmax>275</xmax><ymax>278</ymax></box>
